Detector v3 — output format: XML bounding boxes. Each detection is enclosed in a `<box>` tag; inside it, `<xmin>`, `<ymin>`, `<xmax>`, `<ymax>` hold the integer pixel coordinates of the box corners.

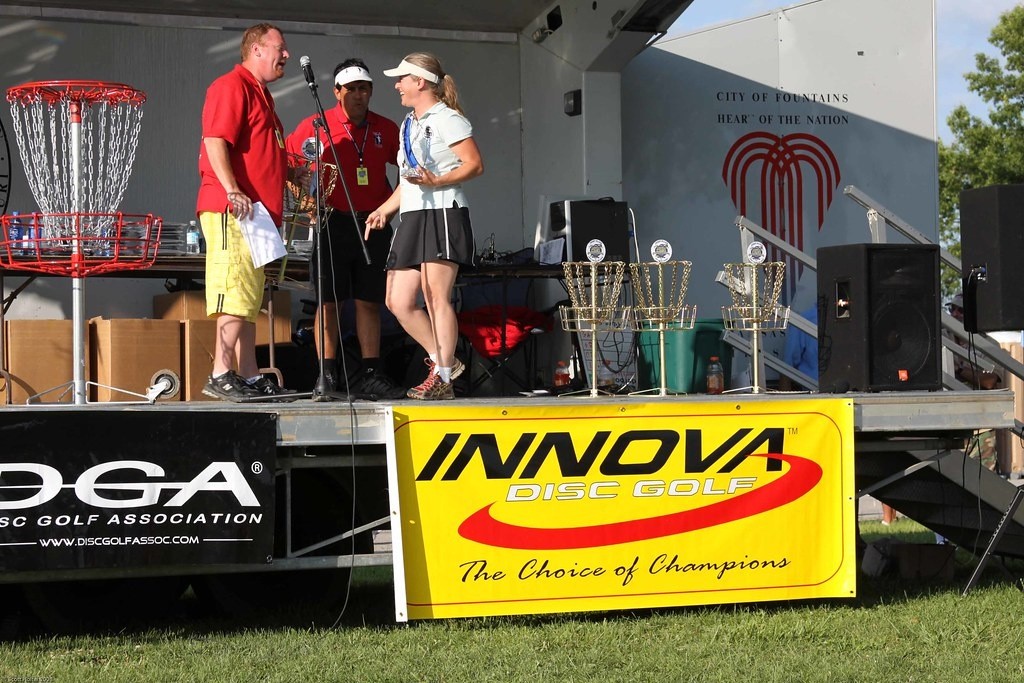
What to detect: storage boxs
<box><xmin>637</xmin><ymin>317</ymin><xmax>734</xmax><ymax>394</ymax></box>
<box><xmin>0</xmin><ymin>290</ymin><xmax>291</xmax><ymax>406</ymax></box>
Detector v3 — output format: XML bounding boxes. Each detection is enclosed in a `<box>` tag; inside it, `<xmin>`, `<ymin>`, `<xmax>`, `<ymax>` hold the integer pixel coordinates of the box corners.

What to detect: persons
<box><xmin>878</xmin><ymin>501</ymin><xmax>900</xmax><ymax>527</ymax></box>
<box><xmin>932</xmin><ymin>292</ymin><xmax>1002</xmax><ymax>555</ymax></box>
<box><xmin>778</xmin><ymin>299</ymin><xmax>821</xmax><ymax>393</ymax></box>
<box><xmin>285</xmin><ymin>58</ymin><xmax>409</xmax><ymax>404</ymax></box>
<box><xmin>364</xmin><ymin>50</ymin><xmax>488</xmax><ymax>400</ymax></box>
<box><xmin>191</xmin><ymin>21</ymin><xmax>302</xmax><ymax>404</ymax></box>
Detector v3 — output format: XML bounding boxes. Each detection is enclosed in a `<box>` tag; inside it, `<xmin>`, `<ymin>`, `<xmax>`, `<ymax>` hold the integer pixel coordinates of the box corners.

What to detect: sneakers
<box><xmin>346</xmin><ymin>371</ymin><xmax>406</xmax><ymax>397</ymax></box>
<box><xmin>250</xmin><ymin>378</ymin><xmax>298</xmax><ymax>401</ymax></box>
<box><xmin>407</xmin><ymin>371</ymin><xmax>455</xmax><ymax>400</ymax></box>
<box><xmin>315</xmin><ymin>370</ymin><xmax>338</xmax><ymax>391</ymax></box>
<box><xmin>424</xmin><ymin>356</ymin><xmax>465</xmax><ymax>380</ymax></box>
<box><xmin>201</xmin><ymin>369</ymin><xmax>260</xmax><ymax>401</ymax></box>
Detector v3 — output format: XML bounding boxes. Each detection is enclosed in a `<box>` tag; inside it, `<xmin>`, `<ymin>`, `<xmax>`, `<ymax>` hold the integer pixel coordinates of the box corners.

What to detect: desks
<box><xmin>0</xmin><ymin>256</ymin><xmax>312</xmax><ymax>404</ymax></box>
<box><xmin>454</xmin><ymin>266</ymin><xmax>643</xmax><ymax>396</ymax></box>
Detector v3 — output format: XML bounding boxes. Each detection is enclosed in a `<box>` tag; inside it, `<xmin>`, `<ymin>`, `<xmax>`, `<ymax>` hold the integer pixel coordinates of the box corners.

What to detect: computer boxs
<box><xmin>549</xmin><ymin>201</ymin><xmax>630</xmax><ymax>266</ymax></box>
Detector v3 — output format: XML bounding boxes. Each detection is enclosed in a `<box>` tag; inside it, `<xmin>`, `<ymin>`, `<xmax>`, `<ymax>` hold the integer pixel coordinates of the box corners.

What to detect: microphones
<box><xmin>299</xmin><ymin>55</ymin><xmax>317</xmax><ymax>99</ymax></box>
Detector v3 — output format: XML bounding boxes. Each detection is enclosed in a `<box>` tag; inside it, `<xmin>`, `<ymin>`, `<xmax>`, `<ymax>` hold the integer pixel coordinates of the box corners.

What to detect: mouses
<box><xmin>599</xmin><ymin>196</ymin><xmax>615</xmax><ymax>200</ymax></box>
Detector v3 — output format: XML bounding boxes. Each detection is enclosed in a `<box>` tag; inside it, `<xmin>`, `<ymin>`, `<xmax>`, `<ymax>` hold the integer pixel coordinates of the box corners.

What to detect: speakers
<box><xmin>817</xmin><ymin>243</ymin><xmax>943</xmax><ymax>394</ymax></box>
<box><xmin>959</xmin><ymin>184</ymin><xmax>1024</xmax><ymax>332</ymax></box>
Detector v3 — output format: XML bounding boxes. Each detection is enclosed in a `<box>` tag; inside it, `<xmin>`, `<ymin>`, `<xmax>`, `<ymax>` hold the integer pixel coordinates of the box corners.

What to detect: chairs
<box><xmin>457</xmin><ymin>248</ymin><xmax>558</xmax><ymax>400</ymax></box>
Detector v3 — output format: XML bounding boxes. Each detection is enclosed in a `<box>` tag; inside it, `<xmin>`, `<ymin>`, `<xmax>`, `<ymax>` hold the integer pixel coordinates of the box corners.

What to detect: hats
<box><xmin>382</xmin><ymin>59</ymin><xmax>440</xmax><ymax>84</ymax></box>
<box><xmin>334</xmin><ymin>66</ymin><xmax>373</xmax><ymax>86</ymax></box>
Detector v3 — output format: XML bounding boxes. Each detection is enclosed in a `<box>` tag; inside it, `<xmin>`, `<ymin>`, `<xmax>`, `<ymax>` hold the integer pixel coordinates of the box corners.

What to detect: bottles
<box><xmin>95</xmin><ymin>216</ymin><xmax>110</xmax><ymax>257</ymax></box>
<box><xmin>554</xmin><ymin>361</ymin><xmax>570</xmax><ymax>386</ymax></box>
<box><xmin>599</xmin><ymin>360</ymin><xmax>614</xmax><ymax>386</ymax></box>
<box><xmin>186</xmin><ymin>220</ymin><xmax>200</xmax><ymax>256</ymax></box>
<box><xmin>9</xmin><ymin>211</ymin><xmax>24</xmax><ymax>256</ymax></box>
<box><xmin>28</xmin><ymin>211</ymin><xmax>41</xmax><ymax>255</ymax></box>
<box><xmin>706</xmin><ymin>357</ymin><xmax>724</xmax><ymax>395</ymax></box>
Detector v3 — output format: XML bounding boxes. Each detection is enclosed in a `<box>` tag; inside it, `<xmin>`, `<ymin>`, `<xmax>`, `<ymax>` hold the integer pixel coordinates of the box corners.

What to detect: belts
<box><xmin>333</xmin><ymin>211</ymin><xmax>368</xmax><ymax>220</ymax></box>
